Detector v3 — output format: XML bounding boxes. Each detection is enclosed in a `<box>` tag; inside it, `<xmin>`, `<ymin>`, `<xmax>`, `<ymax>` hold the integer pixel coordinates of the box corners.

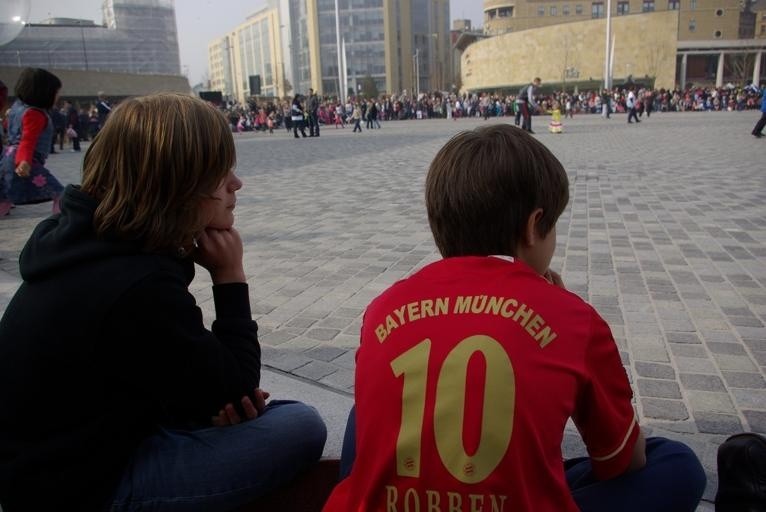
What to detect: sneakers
<box><xmin>295</xmin><ymin>133</ymin><xmax>319</xmax><ymax>137</ymax></box>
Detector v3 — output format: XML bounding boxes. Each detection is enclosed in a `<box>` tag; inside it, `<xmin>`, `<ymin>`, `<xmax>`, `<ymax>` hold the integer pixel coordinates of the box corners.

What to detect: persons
<box><xmin>0</xmin><ymin>92</ymin><xmax>329</xmax><ymax>512</ymax></box>
<box><xmin>751</xmin><ymin>84</ymin><xmax>766</xmax><ymax>137</ymax></box>
<box><xmin>517</xmin><ymin>77</ymin><xmax>542</xmax><ymax>133</ymax></box>
<box><xmin>47</xmin><ymin>92</ymin><xmax>112</xmax><ymax>157</ymax></box>
<box><xmin>0</xmin><ymin>66</ymin><xmax>65</xmax><ymax>219</ymax></box>
<box><xmin>528</xmin><ymin>88</ymin><xmax>761</xmax><ymax>124</ymax></box>
<box><xmin>315</xmin><ymin>122</ymin><xmax>706</xmax><ymax>511</ymax></box>
<box><xmin>218</xmin><ymin>88</ymin><xmax>517</xmax><ymax>139</ymax></box>
